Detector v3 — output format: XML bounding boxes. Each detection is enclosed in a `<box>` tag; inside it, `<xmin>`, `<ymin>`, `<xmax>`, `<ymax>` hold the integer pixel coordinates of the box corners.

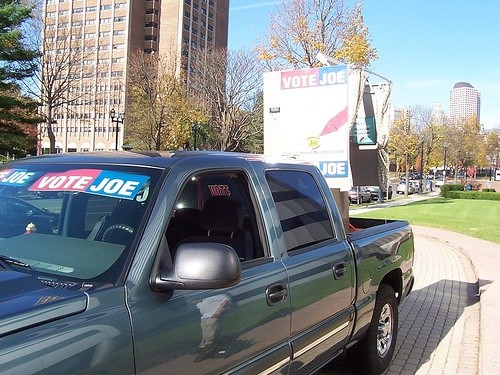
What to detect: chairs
<box><xmin>95</xmin><ymin>199</ymin><xmax>143</xmax><ymax>241</ymax></box>
<box><xmin>171</xmin><ymin>198</ymin><xmax>241</xmax><ymax>268</ymax></box>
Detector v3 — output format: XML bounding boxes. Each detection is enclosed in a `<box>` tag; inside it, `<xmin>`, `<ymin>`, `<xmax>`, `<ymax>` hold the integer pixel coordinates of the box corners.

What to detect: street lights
<box><xmin>110</xmin><ymin>108</ymin><xmax>126</xmax><ymax>152</ymax></box>
<box><xmin>191</xmin><ymin>119</ymin><xmax>201</xmax><ymax>151</ymax></box>
<box><xmin>444</xmin><ymin>146</ymin><xmax>448</xmax><ymax>170</ymax></box>
<box><xmin>454</xmin><ymin>148</ymin><xmax>461</xmax><ymax>177</ymax></box>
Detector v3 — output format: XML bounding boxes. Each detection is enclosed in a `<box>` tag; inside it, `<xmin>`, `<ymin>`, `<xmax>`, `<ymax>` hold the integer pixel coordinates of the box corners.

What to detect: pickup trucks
<box><xmin>0</xmin><ymin>150</ymin><xmax>415</xmax><ymax>375</ymax></box>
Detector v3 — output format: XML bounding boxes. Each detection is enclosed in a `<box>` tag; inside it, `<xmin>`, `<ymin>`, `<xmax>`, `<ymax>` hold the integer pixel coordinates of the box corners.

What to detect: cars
<box><xmin>348</xmin><ymin>185</ymin><xmax>393</xmax><ymax>204</ymax></box>
<box><xmin>465</xmin><ymin>184</ymin><xmax>473</xmax><ymax>190</ymax></box>
<box><xmin>400</xmin><ymin>167</ymin><xmax>500</xmax><ymax>180</ymax></box>
<box><xmin>396</xmin><ymin>180</ymin><xmax>434</xmax><ymax>196</ymax></box>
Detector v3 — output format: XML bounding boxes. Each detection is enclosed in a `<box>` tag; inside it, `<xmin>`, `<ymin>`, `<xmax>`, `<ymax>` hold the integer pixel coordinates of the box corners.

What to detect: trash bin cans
<box><xmin>426</xmin><ymin>181</ymin><xmax>432</xmax><ymax>191</ymax></box>
<box><xmin>466</xmin><ymin>183</ymin><xmax>472</xmax><ymax>191</ymax></box>
